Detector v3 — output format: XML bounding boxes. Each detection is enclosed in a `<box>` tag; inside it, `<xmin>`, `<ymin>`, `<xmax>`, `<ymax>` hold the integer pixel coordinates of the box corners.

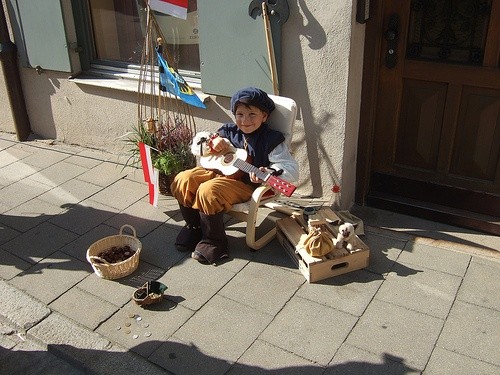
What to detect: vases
<box><xmin>158</xmin><ymin>170</ymin><xmax>176</xmax><ymax>196</ymax></box>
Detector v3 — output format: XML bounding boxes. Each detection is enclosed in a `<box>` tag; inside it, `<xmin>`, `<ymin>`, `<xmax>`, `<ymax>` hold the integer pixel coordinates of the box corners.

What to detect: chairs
<box><xmin>229</xmin><ymin>94</ymin><xmax>297</xmax><ymax>251</ymax></box>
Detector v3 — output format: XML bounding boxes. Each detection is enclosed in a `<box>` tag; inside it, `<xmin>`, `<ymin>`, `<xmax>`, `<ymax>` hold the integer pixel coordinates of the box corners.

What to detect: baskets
<box><xmin>133</xmin><ymin>281</ymin><xmax>165</xmax><ymax>305</ymax></box>
<box><xmin>85</xmin><ymin>224</ymin><xmax>142</xmax><ymax>281</ymax></box>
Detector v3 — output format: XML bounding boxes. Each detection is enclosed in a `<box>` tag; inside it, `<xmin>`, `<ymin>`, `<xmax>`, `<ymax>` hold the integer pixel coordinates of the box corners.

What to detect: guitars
<box><xmin>200</xmin><ymin>137</ymin><xmax>296</xmax><ymax>198</ymax></box>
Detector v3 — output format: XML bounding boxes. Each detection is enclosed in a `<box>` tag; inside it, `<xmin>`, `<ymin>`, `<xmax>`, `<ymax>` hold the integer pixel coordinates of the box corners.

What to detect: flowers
<box><xmin>113</xmin><ymin>123</ymin><xmax>196</xmax><ymax>176</ymax></box>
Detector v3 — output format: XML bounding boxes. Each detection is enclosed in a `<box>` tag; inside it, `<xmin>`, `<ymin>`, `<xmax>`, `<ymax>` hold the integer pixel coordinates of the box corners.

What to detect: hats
<box><xmin>231</xmin><ymin>87</ymin><xmax>276</xmax><ymax>114</ymax></box>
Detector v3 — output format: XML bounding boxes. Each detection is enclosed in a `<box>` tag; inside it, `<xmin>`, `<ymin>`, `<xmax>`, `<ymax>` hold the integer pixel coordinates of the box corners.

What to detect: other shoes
<box><xmin>191</xmin><ymin>248</ymin><xmax>206</xmax><ymax>261</ymax></box>
<box><xmin>175</xmin><ymin>244</ymin><xmax>189</xmax><ymax>251</ymax></box>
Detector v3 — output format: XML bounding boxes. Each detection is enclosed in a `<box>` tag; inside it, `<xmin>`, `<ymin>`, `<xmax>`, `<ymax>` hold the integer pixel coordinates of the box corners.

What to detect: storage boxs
<box><xmin>276</xmin><ymin>208</ymin><xmax>370</xmax><ymax>283</ymax></box>
